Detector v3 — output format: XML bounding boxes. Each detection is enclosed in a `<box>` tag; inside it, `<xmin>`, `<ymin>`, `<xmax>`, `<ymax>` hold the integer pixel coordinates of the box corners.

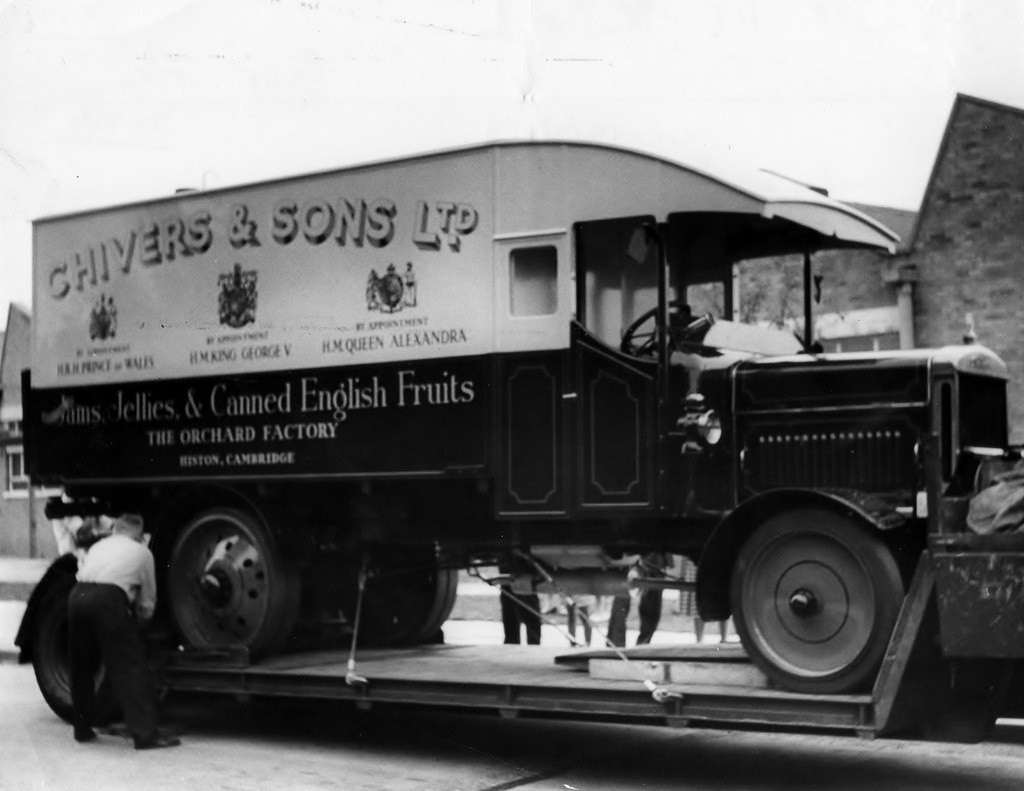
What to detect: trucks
<box><xmin>13</xmin><ymin>92</ymin><xmax>1023</xmax><ymax>746</ymax></box>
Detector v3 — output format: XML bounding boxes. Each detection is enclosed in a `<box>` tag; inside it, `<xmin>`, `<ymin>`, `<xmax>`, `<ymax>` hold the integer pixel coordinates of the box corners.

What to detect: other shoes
<box><xmin>134</xmin><ymin>733</ymin><xmax>180</xmax><ymax>748</ymax></box>
<box><xmin>75</xmin><ymin>729</ymin><xmax>94</xmax><ymax>741</ymax></box>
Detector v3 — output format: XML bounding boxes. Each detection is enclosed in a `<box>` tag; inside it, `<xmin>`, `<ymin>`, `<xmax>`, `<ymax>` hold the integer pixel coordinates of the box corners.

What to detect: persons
<box><xmin>566</xmin><ymin>594</ymin><xmax>594</xmax><ymax>647</ymax></box>
<box><xmin>67</xmin><ymin>511</ymin><xmax>181</xmax><ymax>750</ymax></box>
<box><xmin>695</xmin><ymin>607</ymin><xmax>727</xmax><ymax>646</ymax></box>
<box><xmin>500</xmin><ymin>570</ymin><xmax>541</xmax><ymax>645</ymax></box>
<box><xmin>637</xmin><ymin>552</ymin><xmax>686</xmax><ymax>647</ymax></box>
